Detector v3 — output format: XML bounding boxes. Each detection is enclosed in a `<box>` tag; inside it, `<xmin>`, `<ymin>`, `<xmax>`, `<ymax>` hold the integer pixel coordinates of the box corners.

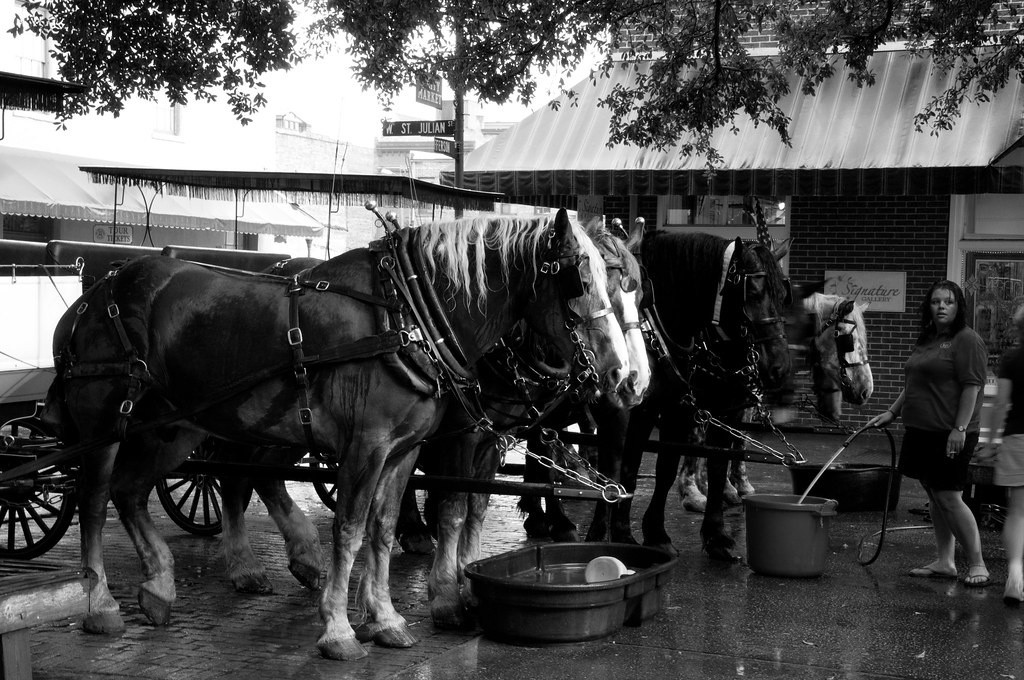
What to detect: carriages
<box><xmin>0</xmin><ymin>205</ymin><xmax>879</xmax><ymax>668</ymax></box>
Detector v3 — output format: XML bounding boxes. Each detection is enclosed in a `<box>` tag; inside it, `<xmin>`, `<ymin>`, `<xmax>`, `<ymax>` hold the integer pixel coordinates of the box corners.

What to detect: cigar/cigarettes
<box><xmin>948</xmin><ymin>451</ymin><xmax>958</xmax><ymax>454</ymax></box>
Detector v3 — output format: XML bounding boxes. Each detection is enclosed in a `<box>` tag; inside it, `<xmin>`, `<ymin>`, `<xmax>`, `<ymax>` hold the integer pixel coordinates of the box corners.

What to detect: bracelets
<box><xmin>887</xmin><ymin>409</ymin><xmax>897</xmax><ymax>421</ymax></box>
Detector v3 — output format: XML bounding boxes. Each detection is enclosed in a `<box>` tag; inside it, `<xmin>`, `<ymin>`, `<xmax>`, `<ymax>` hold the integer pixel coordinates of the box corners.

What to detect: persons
<box><xmin>864</xmin><ymin>278</ymin><xmax>992</xmax><ymax>582</ymax></box>
<box><xmin>979</xmin><ymin>304</ymin><xmax>1024</xmax><ymax>605</ymax></box>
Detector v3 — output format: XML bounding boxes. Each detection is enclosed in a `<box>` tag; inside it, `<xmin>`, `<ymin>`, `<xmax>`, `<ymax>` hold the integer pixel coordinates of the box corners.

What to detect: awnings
<box><xmin>0</xmin><ymin>146</ymin><xmax>325</xmax><ymax>238</ymax></box>
<box><xmin>440</xmin><ymin>42</ymin><xmax>1022</xmax><ymax>198</ymax></box>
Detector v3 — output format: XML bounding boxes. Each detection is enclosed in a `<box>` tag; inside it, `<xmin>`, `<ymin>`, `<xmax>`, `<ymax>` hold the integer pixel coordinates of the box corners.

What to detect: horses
<box><xmin>519</xmin><ymin>231</ymin><xmax>876</xmax><ymax>562</ymax></box>
<box><xmin>41</xmin><ymin>208</ymin><xmax>651</xmax><ymax>660</ymax></box>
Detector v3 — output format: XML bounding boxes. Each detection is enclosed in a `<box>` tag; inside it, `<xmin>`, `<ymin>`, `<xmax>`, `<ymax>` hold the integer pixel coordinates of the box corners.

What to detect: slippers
<box><xmin>964</xmin><ymin>573</ymin><xmax>993</xmax><ymax>587</ymax></box>
<box><xmin>909</xmin><ymin>566</ymin><xmax>959</xmax><ymax>579</ymax></box>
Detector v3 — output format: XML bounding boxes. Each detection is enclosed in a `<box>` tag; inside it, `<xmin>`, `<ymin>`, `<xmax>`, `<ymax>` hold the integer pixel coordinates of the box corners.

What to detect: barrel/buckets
<box><xmin>739</xmin><ymin>491</ymin><xmax>837</xmax><ymax>579</ymax></box>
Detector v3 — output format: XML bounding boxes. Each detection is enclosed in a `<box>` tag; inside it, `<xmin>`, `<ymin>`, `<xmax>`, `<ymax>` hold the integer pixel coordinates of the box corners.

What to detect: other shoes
<box><xmin>1001</xmin><ymin>576</ymin><xmax>1023</xmax><ymax>608</ymax></box>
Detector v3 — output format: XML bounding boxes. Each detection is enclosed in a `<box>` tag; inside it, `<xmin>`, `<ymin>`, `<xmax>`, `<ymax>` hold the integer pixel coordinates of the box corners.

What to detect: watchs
<box><xmin>953</xmin><ymin>425</ymin><xmax>967</xmax><ymax>432</ymax></box>
